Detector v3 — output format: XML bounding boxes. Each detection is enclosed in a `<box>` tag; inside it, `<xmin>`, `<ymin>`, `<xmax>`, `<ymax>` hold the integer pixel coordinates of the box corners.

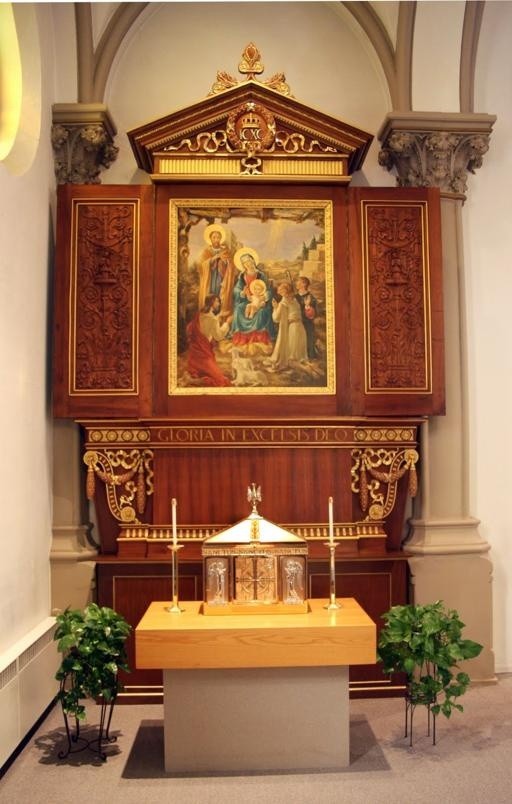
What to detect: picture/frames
<box><xmin>168</xmin><ymin>197</ymin><xmax>338</xmax><ymax>396</ymax></box>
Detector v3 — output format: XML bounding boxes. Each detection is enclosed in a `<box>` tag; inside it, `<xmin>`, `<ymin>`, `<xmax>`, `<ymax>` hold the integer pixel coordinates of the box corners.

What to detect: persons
<box><xmin>178</xmin><ymin>293</ymin><xmax>235</xmax><ymax>388</ymax></box>
<box><xmin>192</xmin><ymin>224</ymin><xmax>236</xmax><ymax>327</ymax></box>
<box><xmin>261</xmin><ymin>282</ymin><xmax>326</xmax><ymax>383</ymax></box>
<box><xmin>243</xmin><ymin>279</ymin><xmax>268</xmax><ymax>320</ymax></box>
<box><xmin>294</xmin><ymin>276</ymin><xmax>321</xmax><ymax>360</ymax></box>
<box><xmin>227</xmin><ymin>247</ymin><xmax>279</xmax><ymax>355</ymax></box>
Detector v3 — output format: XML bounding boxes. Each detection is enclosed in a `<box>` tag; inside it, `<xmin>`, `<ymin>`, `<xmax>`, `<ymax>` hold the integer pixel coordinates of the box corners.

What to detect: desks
<box><xmin>133</xmin><ymin>593</ymin><xmax>380</xmax><ymax>776</ymax></box>
